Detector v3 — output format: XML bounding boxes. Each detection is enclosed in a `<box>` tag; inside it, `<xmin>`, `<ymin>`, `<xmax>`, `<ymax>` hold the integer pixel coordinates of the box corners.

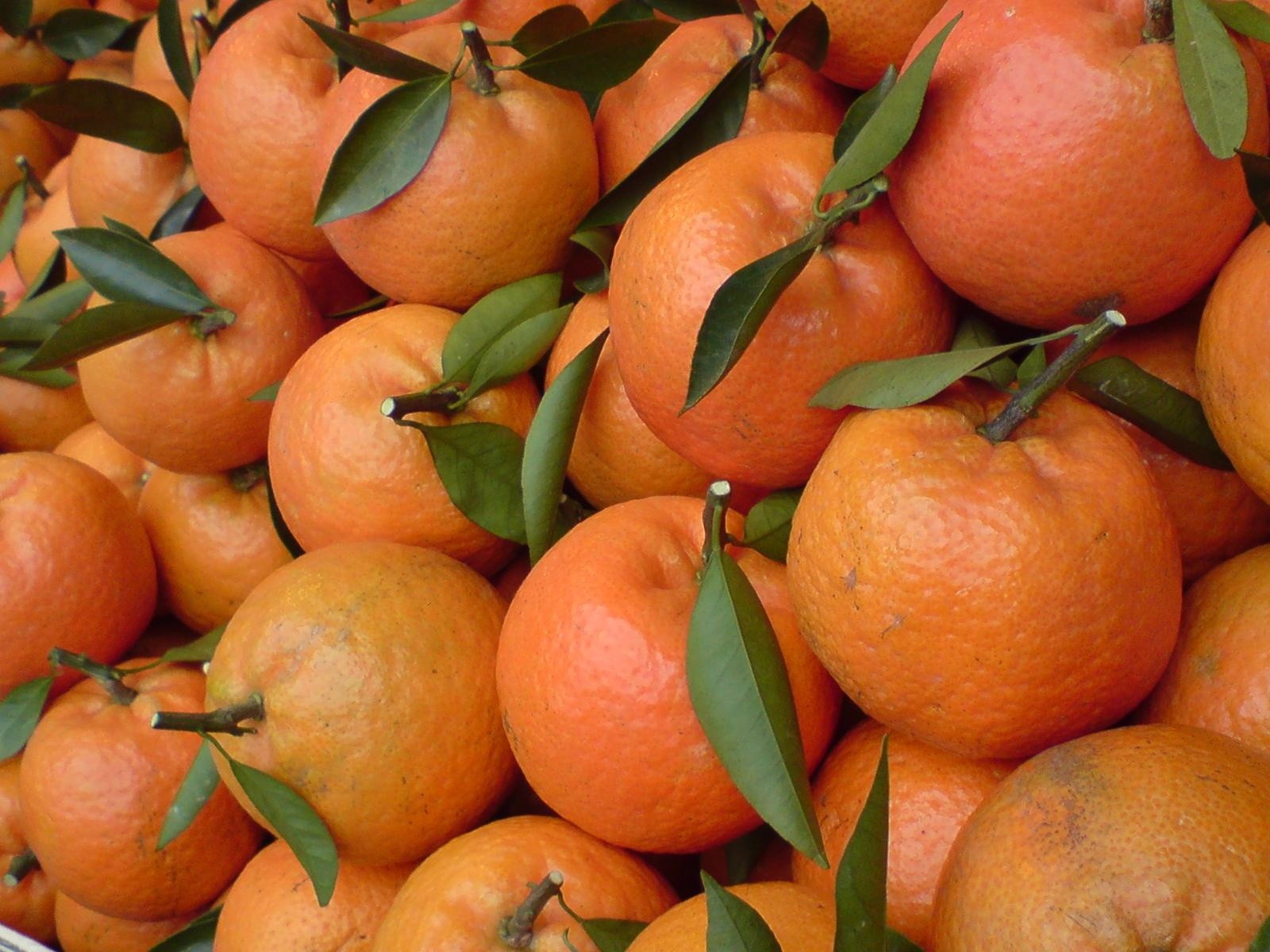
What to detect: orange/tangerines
<box><xmin>0</xmin><ymin>0</ymin><xmax>1267</xmax><ymax>950</ymax></box>
